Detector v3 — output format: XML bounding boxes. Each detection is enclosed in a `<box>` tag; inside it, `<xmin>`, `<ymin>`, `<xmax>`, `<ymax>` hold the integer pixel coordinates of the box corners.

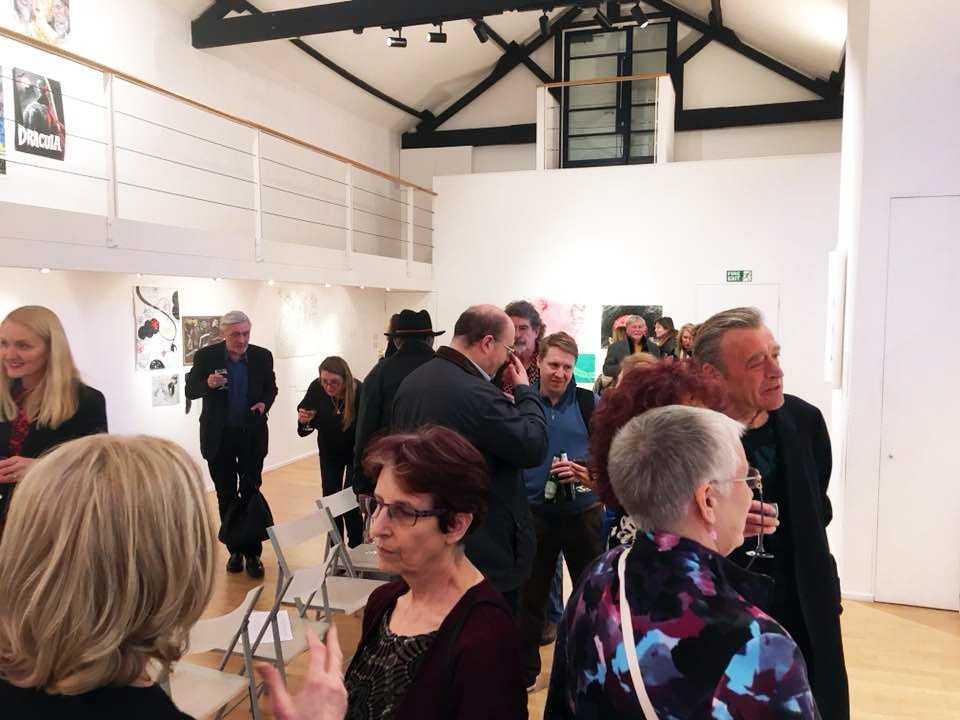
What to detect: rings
<box><xmin>12</xmin><ymin>473</ymin><xmax>16</xmax><ymax>479</ymax></box>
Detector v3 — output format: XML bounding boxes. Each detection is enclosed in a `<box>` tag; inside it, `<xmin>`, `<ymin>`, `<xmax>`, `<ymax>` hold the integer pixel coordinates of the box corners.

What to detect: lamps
<box><xmin>540</xmin><ymin>11</ymin><xmax>551</xmax><ymax>37</ymax></box>
<box><xmin>428</xmin><ymin>23</ymin><xmax>447</xmax><ymax>43</ymax></box>
<box><xmin>388</xmin><ymin>29</ymin><xmax>406</xmax><ymax>46</ymax></box>
<box><xmin>473</xmin><ymin>20</ymin><xmax>488</xmax><ymax>42</ymax></box>
<box><xmin>593</xmin><ymin>7</ymin><xmax>612</xmax><ymax>30</ymax></box>
<box><xmin>631</xmin><ymin>2</ymin><xmax>648</xmax><ymax>28</ymax></box>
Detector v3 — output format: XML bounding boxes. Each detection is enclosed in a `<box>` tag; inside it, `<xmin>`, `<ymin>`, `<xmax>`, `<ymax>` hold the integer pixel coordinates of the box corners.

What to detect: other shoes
<box><xmin>538</xmin><ymin>620</ymin><xmax>557</xmax><ymax>645</ymax></box>
<box><xmin>526</xmin><ymin>677</ymin><xmax>538</xmax><ymax>691</ymax></box>
<box><xmin>227</xmin><ymin>552</ymin><xmax>244</xmax><ymax>574</ymax></box>
<box><xmin>246</xmin><ymin>557</ymin><xmax>265</xmax><ymax>579</ymax></box>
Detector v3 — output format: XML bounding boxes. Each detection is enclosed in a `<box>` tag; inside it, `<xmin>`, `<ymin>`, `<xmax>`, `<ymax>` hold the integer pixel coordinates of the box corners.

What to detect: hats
<box><xmin>384</xmin><ymin>309</ymin><xmax>446</xmax><ymax>338</ymax></box>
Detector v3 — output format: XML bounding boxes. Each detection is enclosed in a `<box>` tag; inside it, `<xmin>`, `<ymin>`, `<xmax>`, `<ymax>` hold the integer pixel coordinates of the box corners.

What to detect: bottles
<box><xmin>542</xmin><ymin>454</ymin><xmax>560</xmax><ymax>503</ymax></box>
<box><xmin>560</xmin><ymin>447</ymin><xmax>576</xmax><ymax>501</ymax></box>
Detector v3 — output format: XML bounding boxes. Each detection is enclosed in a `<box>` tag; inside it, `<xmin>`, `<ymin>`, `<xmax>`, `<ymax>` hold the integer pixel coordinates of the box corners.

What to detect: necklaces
<box><xmin>329</xmin><ymin>396</ymin><xmax>346</xmax><ymax>415</ymax></box>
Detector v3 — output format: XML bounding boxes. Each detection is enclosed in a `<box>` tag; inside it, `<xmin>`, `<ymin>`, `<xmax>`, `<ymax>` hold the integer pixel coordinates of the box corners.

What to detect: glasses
<box><xmin>728</xmin><ymin>467</ymin><xmax>762</xmax><ymax>488</ymax></box>
<box><xmin>358</xmin><ymin>493</ymin><xmax>438</xmax><ymax>527</ymax></box>
<box><xmin>319</xmin><ymin>380</ymin><xmax>340</xmax><ymax>389</ymax></box>
<box><xmin>504</xmin><ymin>344</ymin><xmax>520</xmax><ymax>358</ymax></box>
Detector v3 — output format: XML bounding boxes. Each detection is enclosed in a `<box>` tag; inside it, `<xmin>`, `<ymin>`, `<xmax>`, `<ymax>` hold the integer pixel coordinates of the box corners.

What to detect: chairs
<box><xmin>146</xmin><ymin>484</ymin><xmax>392</xmax><ymax>720</ymax></box>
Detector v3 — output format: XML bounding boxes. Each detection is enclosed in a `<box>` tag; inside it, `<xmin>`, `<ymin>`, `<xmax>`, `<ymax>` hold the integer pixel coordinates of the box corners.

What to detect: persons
<box><xmin>693</xmin><ymin>309</ymin><xmax>849</xmax><ymax>720</ymax></box>
<box><xmin>296</xmin><ymin>301</ymin><xmax>736</xmax><ymax>692</ymax></box>
<box><xmin>343</xmin><ymin>423</ymin><xmax>530</xmax><ymax>720</ymax></box>
<box><xmin>544</xmin><ymin>404</ymin><xmax>823</xmax><ymax>720</ymax></box>
<box><xmin>36</xmin><ymin>78</ymin><xmax>55</xmax><ymax>107</ymax></box>
<box><xmin>185</xmin><ymin>311</ymin><xmax>278</xmax><ymax>579</ymax></box>
<box><xmin>0</xmin><ymin>433</ymin><xmax>347</xmax><ymax>720</ymax></box>
<box><xmin>0</xmin><ymin>306</ymin><xmax>108</xmax><ymax>543</ymax></box>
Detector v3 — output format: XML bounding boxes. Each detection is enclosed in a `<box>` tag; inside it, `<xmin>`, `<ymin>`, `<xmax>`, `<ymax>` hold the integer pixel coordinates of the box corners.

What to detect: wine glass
<box><xmin>303</xmin><ymin>410</ymin><xmax>315</xmax><ymax>431</ymax></box>
<box><xmin>214</xmin><ymin>368</ymin><xmax>229</xmax><ymax>390</ymax></box>
<box><xmin>746</xmin><ymin>504</ymin><xmax>778</xmax><ymax>558</ymax></box>
